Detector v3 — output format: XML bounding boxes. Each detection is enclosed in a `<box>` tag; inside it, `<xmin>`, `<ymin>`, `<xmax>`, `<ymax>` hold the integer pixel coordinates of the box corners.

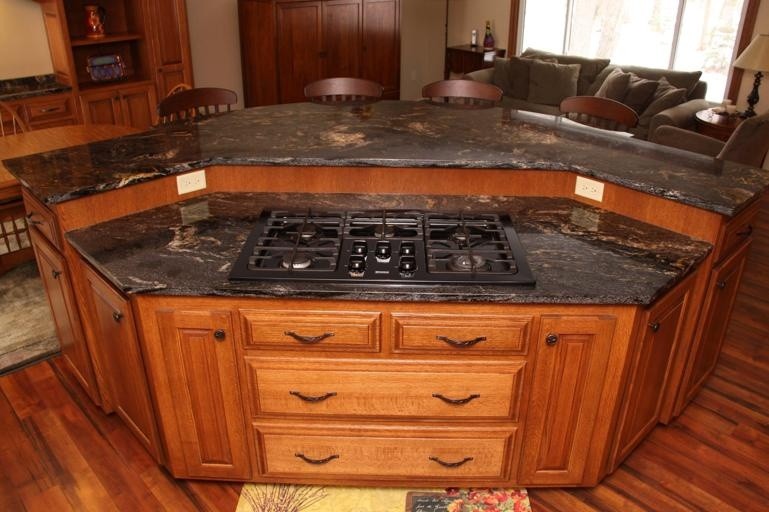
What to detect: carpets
<box><xmin>0</xmin><ymin>208</ymin><xmax>64</xmax><ymax>383</ymax></box>
<box><xmin>222</xmin><ymin>477</ymin><xmax>534</xmax><ymax>511</ymax></box>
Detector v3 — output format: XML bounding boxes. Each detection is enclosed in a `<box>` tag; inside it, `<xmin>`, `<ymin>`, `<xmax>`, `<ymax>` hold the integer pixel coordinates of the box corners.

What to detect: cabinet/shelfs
<box><xmin>510</xmin><ymin>292</ymin><xmax>643</xmax><ymax>497</ymax></box>
<box><xmin>241</xmin><ymin>350</ymin><xmax>522</xmax><ymax>422</ymax></box>
<box><xmin>359</xmin><ymin>0</ymin><xmax>401</xmax><ymax>106</ymax></box>
<box><xmin>20</xmin><ymin>182</ymin><xmax>115</xmax><ymax>421</ymax></box>
<box><xmin>1</xmin><ymin>0</ymin><xmax>198</xmax><ymax>130</ymax></box>
<box><xmin>234</xmin><ymin>0</ymin><xmax>363</xmax><ymax>111</ymax></box>
<box><xmin>241</xmin><ymin>290</ymin><xmax>537</xmax><ymax>362</ymax></box>
<box><xmin>76</xmin><ymin>256</ymin><xmax>167</xmax><ymax>480</ymax></box>
<box><xmin>124</xmin><ymin>290</ymin><xmax>255</xmax><ymax>486</ymax></box>
<box><xmin>244</xmin><ymin>412</ymin><xmax>520</xmax><ymax>488</ymax></box>
<box><xmin>654</xmin><ymin>187</ymin><xmax>769</xmax><ymax>427</ymax></box>
<box><xmin>607</xmin><ymin>261</ymin><xmax>702</xmax><ymax>481</ymax></box>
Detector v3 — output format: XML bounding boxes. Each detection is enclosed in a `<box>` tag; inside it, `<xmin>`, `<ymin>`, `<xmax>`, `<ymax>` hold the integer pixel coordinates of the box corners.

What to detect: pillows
<box><xmin>487</xmin><ymin>51</ymin><xmax>704</xmax><ymax>131</ymax></box>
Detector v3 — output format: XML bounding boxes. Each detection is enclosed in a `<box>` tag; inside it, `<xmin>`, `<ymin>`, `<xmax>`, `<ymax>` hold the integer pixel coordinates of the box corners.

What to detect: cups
<box><xmin>720</xmin><ymin>97</ymin><xmax>738</xmax><ymax>114</ymax></box>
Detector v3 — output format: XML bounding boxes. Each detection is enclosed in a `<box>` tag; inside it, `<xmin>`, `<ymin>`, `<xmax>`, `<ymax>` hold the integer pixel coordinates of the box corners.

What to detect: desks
<box><xmin>443</xmin><ymin>40</ymin><xmax>506</xmax><ymax>105</ymax></box>
<box><xmin>0</xmin><ymin>122</ymin><xmax>151</xmax><ymax>281</ymax></box>
<box><xmin>691</xmin><ymin>106</ymin><xmax>753</xmax><ymax>143</ymax></box>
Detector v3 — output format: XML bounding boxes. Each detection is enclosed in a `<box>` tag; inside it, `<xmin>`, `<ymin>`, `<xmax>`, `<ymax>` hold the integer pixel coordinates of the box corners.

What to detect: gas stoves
<box><xmin>225</xmin><ymin>202</ymin><xmax>535</xmax><ymax>288</ymax></box>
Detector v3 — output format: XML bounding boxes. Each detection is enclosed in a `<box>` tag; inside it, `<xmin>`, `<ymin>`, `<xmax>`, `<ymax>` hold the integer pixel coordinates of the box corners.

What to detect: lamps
<box><xmin>730</xmin><ymin>31</ymin><xmax>769</xmax><ymax>122</ymax></box>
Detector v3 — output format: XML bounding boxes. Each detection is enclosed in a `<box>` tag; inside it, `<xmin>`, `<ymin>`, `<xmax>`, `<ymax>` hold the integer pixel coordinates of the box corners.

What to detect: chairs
<box><xmin>559</xmin><ymin>95</ymin><xmax>641</xmax><ymax>134</ymax></box>
<box><xmin>647</xmin><ymin>105</ymin><xmax>768</xmax><ymax>175</ymax></box>
<box><xmin>152</xmin><ymin>86</ymin><xmax>239</xmax><ymax>125</ymax></box>
<box><xmin>420</xmin><ymin>79</ymin><xmax>505</xmax><ymax>109</ymax></box>
<box><xmin>302</xmin><ymin>77</ymin><xmax>385</xmax><ymax>103</ymax></box>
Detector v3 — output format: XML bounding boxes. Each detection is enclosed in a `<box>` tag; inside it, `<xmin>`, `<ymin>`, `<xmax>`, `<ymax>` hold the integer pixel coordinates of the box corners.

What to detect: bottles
<box><xmin>471</xmin><ymin>31</ymin><xmax>476</xmax><ymax>46</ymax></box>
<box><xmin>484</xmin><ymin>21</ymin><xmax>495</xmax><ymax>51</ymax></box>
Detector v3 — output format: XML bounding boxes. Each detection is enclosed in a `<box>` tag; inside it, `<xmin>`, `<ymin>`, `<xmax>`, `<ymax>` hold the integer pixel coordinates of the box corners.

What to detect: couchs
<box><xmin>458</xmin><ymin>48</ymin><xmax>712</xmax><ymax>147</ymax></box>
